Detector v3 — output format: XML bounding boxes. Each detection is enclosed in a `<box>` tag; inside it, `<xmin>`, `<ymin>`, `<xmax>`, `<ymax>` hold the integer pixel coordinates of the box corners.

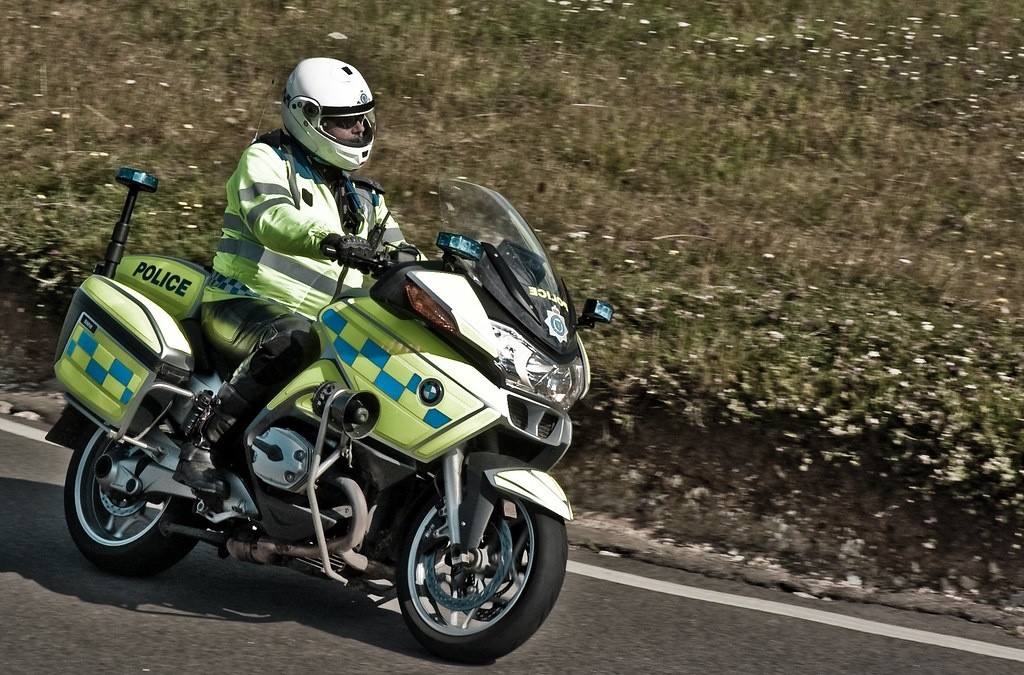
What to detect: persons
<box><xmin>171</xmin><ymin>58</ymin><xmax>428</xmax><ymax>499</ymax></box>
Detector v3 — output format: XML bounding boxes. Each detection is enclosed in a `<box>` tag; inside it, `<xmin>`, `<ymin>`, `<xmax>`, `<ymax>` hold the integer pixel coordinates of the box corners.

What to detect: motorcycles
<box><xmin>45</xmin><ymin>167</ymin><xmax>616</xmax><ymax>666</ymax></box>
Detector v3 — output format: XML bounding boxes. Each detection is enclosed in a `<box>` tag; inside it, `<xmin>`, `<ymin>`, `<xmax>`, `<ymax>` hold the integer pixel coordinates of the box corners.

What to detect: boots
<box><xmin>171</xmin><ymin>380</ymin><xmax>250</xmax><ymax>500</ymax></box>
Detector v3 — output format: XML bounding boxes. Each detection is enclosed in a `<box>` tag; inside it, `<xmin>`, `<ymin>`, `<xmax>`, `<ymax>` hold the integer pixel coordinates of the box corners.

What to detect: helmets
<box><xmin>280</xmin><ymin>57</ymin><xmax>376</xmax><ymax>171</ymax></box>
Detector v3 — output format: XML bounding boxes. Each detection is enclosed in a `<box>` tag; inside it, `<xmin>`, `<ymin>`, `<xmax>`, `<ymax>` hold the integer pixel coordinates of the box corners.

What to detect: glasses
<box><xmin>322</xmin><ymin>115</ymin><xmax>365</xmax><ymax>128</ymax></box>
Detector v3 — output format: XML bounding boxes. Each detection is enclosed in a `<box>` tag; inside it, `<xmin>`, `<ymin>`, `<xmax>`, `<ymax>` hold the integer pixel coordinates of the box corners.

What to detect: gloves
<box><xmin>321</xmin><ymin>232</ymin><xmax>372</xmax><ymax>276</ymax></box>
